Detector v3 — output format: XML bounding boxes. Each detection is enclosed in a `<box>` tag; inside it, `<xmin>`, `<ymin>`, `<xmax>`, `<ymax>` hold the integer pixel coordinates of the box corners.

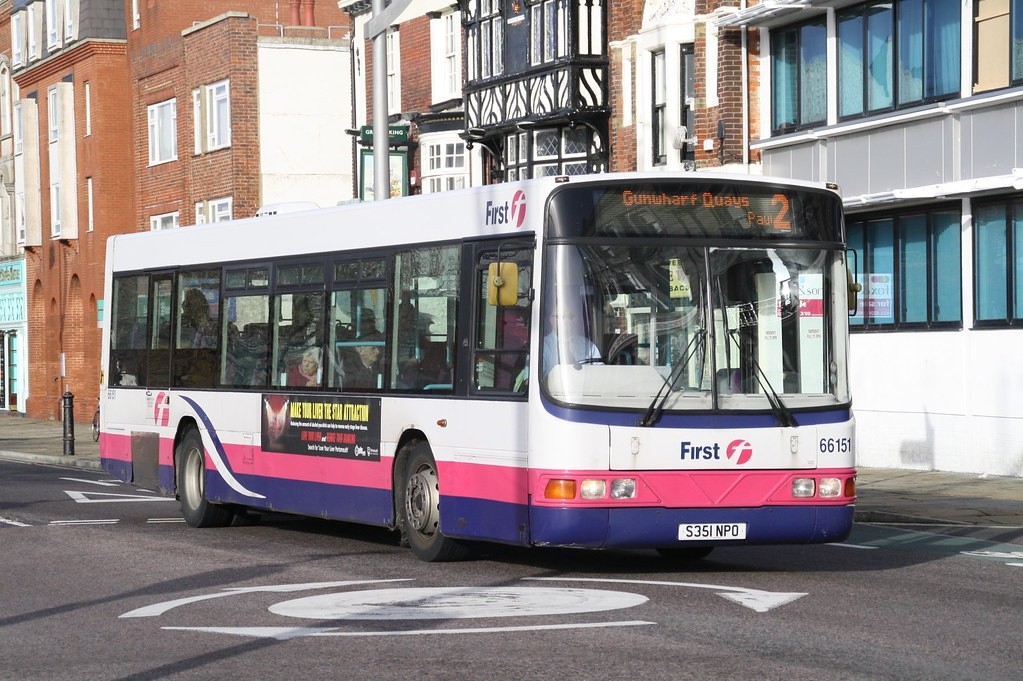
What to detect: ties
<box><xmin>563</xmin><ymin>341</ymin><xmax>576</xmax><ymax>365</ymax></box>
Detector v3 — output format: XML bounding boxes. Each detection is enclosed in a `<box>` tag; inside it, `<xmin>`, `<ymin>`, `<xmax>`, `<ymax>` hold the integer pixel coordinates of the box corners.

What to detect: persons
<box><xmin>335</xmin><ymin>336</ymin><xmax>383</xmax><ymax>388</ymax></box>
<box><xmin>349</xmin><ymin>307</ymin><xmax>382</xmax><ymax>343</ymax></box>
<box><xmin>524</xmin><ymin>299</ymin><xmax>603</xmax><ymax>385</ymax></box>
<box><xmin>286</xmin><ymin>346</ymin><xmax>323</xmax><ymax>388</ymax></box>
<box><xmin>153</xmin><ymin>289</ymin><xmax>211</xmax><ymax>386</ymax></box>
<box><xmin>399</xmin><ymin>302</ymin><xmax>445</xmax><ymax>389</ymax></box>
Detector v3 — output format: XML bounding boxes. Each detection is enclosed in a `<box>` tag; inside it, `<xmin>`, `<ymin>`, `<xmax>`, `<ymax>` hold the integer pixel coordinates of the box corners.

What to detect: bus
<box><xmin>100</xmin><ymin>170</ymin><xmax>862</xmax><ymax>562</ymax></box>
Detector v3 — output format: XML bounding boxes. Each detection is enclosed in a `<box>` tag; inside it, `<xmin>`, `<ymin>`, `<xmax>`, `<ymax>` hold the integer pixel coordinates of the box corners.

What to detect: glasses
<box><xmin>549</xmin><ymin>314</ymin><xmax>576</xmax><ymax>320</ymax></box>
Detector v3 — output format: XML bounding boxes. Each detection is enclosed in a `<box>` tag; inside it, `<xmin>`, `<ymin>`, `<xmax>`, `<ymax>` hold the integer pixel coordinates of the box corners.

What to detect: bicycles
<box><xmin>91</xmin><ymin>398</ymin><xmax>100</xmax><ymax>442</ymax></box>
